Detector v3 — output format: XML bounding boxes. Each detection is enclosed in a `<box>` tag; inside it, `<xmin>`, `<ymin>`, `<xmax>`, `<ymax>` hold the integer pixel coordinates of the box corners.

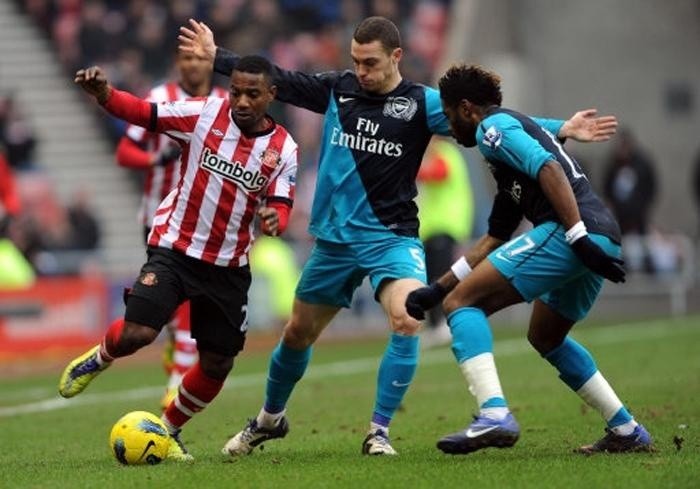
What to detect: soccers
<box><xmin>111</xmin><ymin>411</ymin><xmax>169</xmax><ymax>464</ymax></box>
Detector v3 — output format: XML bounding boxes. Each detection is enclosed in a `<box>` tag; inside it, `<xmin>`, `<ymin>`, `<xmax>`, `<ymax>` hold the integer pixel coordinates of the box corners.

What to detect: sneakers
<box><xmin>161</xmin><ymin>390</ymin><xmax>180</xmax><ymax>408</ymax></box>
<box><xmin>59</xmin><ymin>345</ymin><xmax>113</xmax><ymax>398</ymax></box>
<box><xmin>167</xmin><ymin>433</ymin><xmax>195</xmax><ymax>461</ymax></box>
<box><xmin>578</xmin><ymin>423</ymin><xmax>653</xmax><ymax>455</ymax></box>
<box><xmin>221</xmin><ymin>417</ymin><xmax>288</xmax><ymax>456</ymax></box>
<box><xmin>437</xmin><ymin>413</ymin><xmax>519</xmax><ymax>455</ymax></box>
<box><xmin>364</xmin><ymin>432</ymin><xmax>396</xmax><ymax>456</ymax></box>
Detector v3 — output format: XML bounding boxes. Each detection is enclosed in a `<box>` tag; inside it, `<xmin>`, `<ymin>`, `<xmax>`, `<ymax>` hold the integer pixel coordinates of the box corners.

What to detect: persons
<box><xmin>115</xmin><ymin>43</ymin><xmax>232</xmax><ymax>417</ymax></box>
<box><xmin>0</xmin><ymin>1</ymin><xmax>699</xmax><ymax>288</ymax></box>
<box><xmin>405</xmin><ymin>65</ymin><xmax>656</xmax><ymax>453</ymax></box>
<box><xmin>176</xmin><ymin>17</ymin><xmax>619</xmax><ymax>458</ymax></box>
<box><xmin>59</xmin><ymin>54</ymin><xmax>299</xmax><ymax>462</ymax></box>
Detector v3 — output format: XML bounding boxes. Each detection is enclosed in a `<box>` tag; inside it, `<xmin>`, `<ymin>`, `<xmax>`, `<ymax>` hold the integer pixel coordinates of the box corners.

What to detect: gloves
<box><xmin>406</xmin><ymin>283</ymin><xmax>443</xmax><ymax>321</ymax></box>
<box><xmin>570</xmin><ymin>236</ymin><xmax>626</xmax><ymax>283</ymax></box>
